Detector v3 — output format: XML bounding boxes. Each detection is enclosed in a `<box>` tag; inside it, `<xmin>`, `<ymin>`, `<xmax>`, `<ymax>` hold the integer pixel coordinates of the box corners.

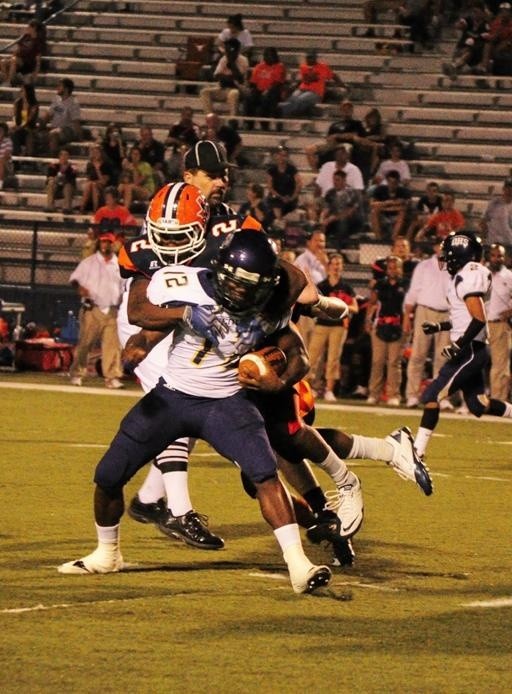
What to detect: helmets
<box><xmin>210</xmin><ymin>227</ymin><xmax>279</xmax><ymax>313</ymax></box>
<box><xmin>148</xmin><ymin>180</ymin><xmax>210</xmax><ymax>265</ymax></box>
<box><xmin>438</xmin><ymin>231</ymin><xmax>484</xmax><ymax>274</ymax></box>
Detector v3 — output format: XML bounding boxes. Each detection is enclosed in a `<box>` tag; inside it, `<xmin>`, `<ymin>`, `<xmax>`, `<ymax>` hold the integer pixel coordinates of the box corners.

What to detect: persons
<box><xmin>365</xmin><ymin>230</ymin><xmax>512</xmax><ymax>414</ymax></box>
<box><xmin>79</xmin><ymin>106</ymin><xmax>243</xmax><ymax>216</ymax></box>
<box><xmin>353</xmin><ymin>108</ymin><xmax>386</xmax><ymax>173</ymax></box>
<box><xmin>479</xmin><ymin>178</ymin><xmax>512</xmax><ymax>245</ymax></box>
<box><xmin>69</xmin><ymin>231</ymin><xmax>125</xmax><ymax>389</ymax></box>
<box><xmin>371</xmin><ymin>169</ymin><xmax>411</xmax><ymax>240</ymax></box>
<box><xmin>375</xmin><ymin>141</ymin><xmax>411</xmax><ymax>187</ymax></box>
<box><xmin>288</xmin><ymin>49</ymin><xmax>350</xmax><ymax>120</ymax></box>
<box><xmin>8</xmin><ymin>84</ymin><xmax>39</xmax><ymax>165</ymax></box>
<box><xmin>213</xmin><ymin>13</ymin><xmax>253</xmax><ymax>61</ymax></box>
<box><xmin>43</xmin><ymin>148</ymin><xmax>75</xmax><ymax>214</ymax></box>
<box><xmin>245</xmin><ymin>47</ymin><xmax>286</xmax><ymax>130</ymax></box>
<box><xmin>413</xmin><ymin>230</ymin><xmax>512</xmax><ymax>462</ymax></box>
<box><xmin>33</xmin><ymin>78</ymin><xmax>83</xmax><ymax>158</ymax></box>
<box><xmin>291</xmin><ymin>230</ymin><xmax>328</xmax><ymax>334</ymax></box>
<box><xmin>238</xmin><ymin>182</ymin><xmax>271</xmax><ymax>227</ymax></box>
<box><xmin>10</xmin><ymin>20</ymin><xmax>47</xmax><ymax>114</ymax></box>
<box><xmin>362</xmin><ymin>0</ymin><xmax>512</xmax><ymax>75</ymax></box>
<box><xmin>306</xmin><ymin>99</ymin><xmax>365</xmax><ymax>174</ymax></box>
<box><xmin>118</xmin><ymin>180</ymin><xmax>434</xmax><ymax>566</ymax></box>
<box><xmin>317</xmin><ymin>171</ymin><xmax>363</xmax><ymax>239</ymax></box>
<box><xmin>291</xmin><ymin>230</ymin><xmax>328</xmax><ymax>341</ymax></box>
<box><xmin>414</xmin><ymin>190</ymin><xmax>464</xmax><ymax>241</ymax></box>
<box><xmin>58</xmin><ymin>229</ymin><xmax>332</xmax><ymax>594</ymax></box>
<box><xmin>116</xmin><ymin>139</ymin><xmax>242</xmax><ymax>550</ymax></box>
<box><xmin>313</xmin><ymin>143</ymin><xmax>363</xmax><ymax>197</ymax></box>
<box><xmin>308</xmin><ymin>252</ymin><xmax>359</xmax><ymax>404</ymax></box>
<box><xmin>0</xmin><ymin>122</ymin><xmax>14</xmax><ymax>190</ymax></box>
<box><xmin>199</xmin><ymin>37</ymin><xmax>249</xmax><ymax>115</ymax></box>
<box><xmin>267</xmin><ymin>148</ymin><xmax>303</xmax><ymax>221</ymax></box>
<box><xmin>406</xmin><ymin>182</ymin><xmax>440</xmax><ymax>240</ymax></box>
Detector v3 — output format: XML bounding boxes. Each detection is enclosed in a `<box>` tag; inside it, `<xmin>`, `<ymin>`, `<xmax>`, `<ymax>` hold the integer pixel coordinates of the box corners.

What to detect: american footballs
<box><xmin>239</xmin><ymin>344</ymin><xmax>288</xmax><ymax>379</ymax></box>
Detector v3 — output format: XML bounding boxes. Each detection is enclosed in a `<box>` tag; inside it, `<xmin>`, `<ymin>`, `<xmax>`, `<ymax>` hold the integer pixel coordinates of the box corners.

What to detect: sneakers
<box><xmin>57</xmin><ymin>548</ymin><xmax>122</xmax><ymax>574</ymax></box>
<box><xmin>456</xmin><ymin>402</ymin><xmax>468</xmax><ymax>413</ymax></box>
<box><xmin>325</xmin><ymin>390</ymin><xmax>334</xmax><ymax>402</ymax></box>
<box><xmin>129</xmin><ymin>493</ymin><xmax>165</xmax><ymax>522</ymax></box>
<box><xmin>388</xmin><ymin>397</ymin><xmax>399</xmax><ymax>407</ymax></box>
<box><xmin>159</xmin><ymin>508</ymin><xmax>225</xmax><ymax>548</ymax></box>
<box><xmin>406</xmin><ymin>397</ymin><xmax>419</xmax><ymax>407</ymax></box>
<box><xmin>105</xmin><ymin>377</ymin><xmax>124</xmax><ymax>388</ymax></box>
<box><xmin>299</xmin><ymin>498</ymin><xmax>322</xmax><ymax>545</ymax></box>
<box><xmin>385</xmin><ymin>426</ymin><xmax>433</xmax><ymax>496</ymax></box>
<box><xmin>289</xmin><ymin>565</ymin><xmax>331</xmax><ymax>593</ymax></box>
<box><xmin>71</xmin><ymin>376</ymin><xmax>82</xmax><ymax>385</ymax></box>
<box><xmin>322</xmin><ymin>512</ymin><xmax>355</xmax><ymax>568</ymax></box>
<box><xmin>442</xmin><ymin>62</ymin><xmax>458</xmax><ymax>80</ymax></box>
<box><xmin>439</xmin><ymin>397</ymin><xmax>452</xmax><ymax>409</ymax></box>
<box><xmin>366</xmin><ymin>396</ymin><xmax>378</xmax><ymax>404</ymax></box>
<box><xmin>324</xmin><ymin>470</ymin><xmax>364</xmax><ymax>539</ymax></box>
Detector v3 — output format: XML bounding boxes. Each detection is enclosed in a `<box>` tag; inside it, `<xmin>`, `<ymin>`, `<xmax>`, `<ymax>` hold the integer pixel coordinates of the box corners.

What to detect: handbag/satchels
<box><xmin>375</xmin><ymin>321</ymin><xmax>400</xmax><ymax>342</ymax></box>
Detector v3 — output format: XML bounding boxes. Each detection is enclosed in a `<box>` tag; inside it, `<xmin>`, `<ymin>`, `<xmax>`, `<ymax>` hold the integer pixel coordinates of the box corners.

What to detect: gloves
<box><xmin>422</xmin><ymin>319</ymin><xmax>440</xmax><ymax>334</ymax></box>
<box><xmin>441</xmin><ymin>337</ymin><xmax>466</xmax><ymax>360</ymax></box>
<box><xmin>184</xmin><ymin>304</ymin><xmax>231</xmax><ymax>348</ymax></box>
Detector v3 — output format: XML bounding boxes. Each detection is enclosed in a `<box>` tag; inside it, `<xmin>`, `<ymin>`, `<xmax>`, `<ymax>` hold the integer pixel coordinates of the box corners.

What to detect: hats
<box><xmin>340</xmin><ymin>99</ymin><xmax>351</xmax><ymax>107</ymax></box>
<box><xmin>99</xmin><ymin>232</ymin><xmax>116</xmax><ymax>242</ymax></box>
<box><xmin>229</xmin><ymin>13</ymin><xmax>245</xmax><ymax>30</ymax></box>
<box><xmin>57</xmin><ymin>77</ymin><xmax>74</xmax><ymax>89</ymax></box>
<box><xmin>271</xmin><ymin>145</ymin><xmax>285</xmax><ymax>150</ymax></box>
<box><xmin>365</xmin><ymin>106</ymin><xmax>379</xmax><ymax>117</ymax></box>
<box><xmin>305</xmin><ymin>50</ymin><xmax>315</xmax><ymax>56</ymax></box>
<box><xmin>185</xmin><ymin>138</ymin><xmax>239</xmax><ymax>171</ymax></box>
